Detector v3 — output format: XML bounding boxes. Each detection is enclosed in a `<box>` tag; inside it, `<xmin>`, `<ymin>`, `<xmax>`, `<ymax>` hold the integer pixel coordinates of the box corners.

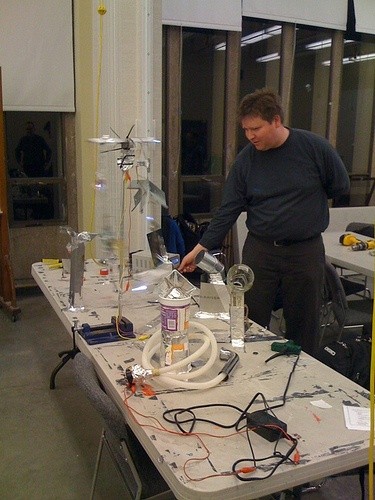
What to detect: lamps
<box><xmin>213</xmin><ymin>24</ymin><xmax>375</xmax><ymax>67</ymax></box>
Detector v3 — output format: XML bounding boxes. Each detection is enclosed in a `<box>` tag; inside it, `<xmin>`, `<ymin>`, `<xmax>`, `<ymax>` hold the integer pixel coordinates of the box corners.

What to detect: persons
<box><xmin>16</xmin><ymin>121</ymin><xmax>52</xmax><ymax>220</ymax></box>
<box><xmin>178</xmin><ymin>86</ymin><xmax>351</xmax><ymax>360</ymax></box>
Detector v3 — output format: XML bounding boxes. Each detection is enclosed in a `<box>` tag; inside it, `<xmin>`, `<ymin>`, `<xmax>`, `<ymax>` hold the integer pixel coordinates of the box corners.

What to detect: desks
<box><xmin>321</xmin><ymin>231</ymin><xmax>375</xmax><ymax>279</ymax></box>
<box><xmin>31</xmin><ymin>257</ymin><xmax>375</xmax><ymax>500</ymax></box>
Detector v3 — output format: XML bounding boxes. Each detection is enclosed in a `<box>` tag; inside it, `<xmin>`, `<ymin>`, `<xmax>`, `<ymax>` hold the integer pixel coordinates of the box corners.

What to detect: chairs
<box><xmin>321</xmin><ymin>222</ymin><xmax>375</xmax><ymax>317</ymax></box>
<box><xmin>318</xmin><ymin>260</ymin><xmax>373</xmax><ymax>346</ymax></box>
<box><xmin>340</xmin><ymin>174</ymin><xmax>375</xmax><ymax>207</ymax></box>
<box><xmin>73</xmin><ymin>351</ymin><xmax>173</xmax><ymax>500</ymax></box>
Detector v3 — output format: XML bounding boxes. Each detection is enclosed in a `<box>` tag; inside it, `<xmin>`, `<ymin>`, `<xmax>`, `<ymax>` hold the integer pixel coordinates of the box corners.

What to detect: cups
<box><xmin>158</xmin><ymin>294</ymin><xmax>191</xmax><ymax>332</ymax></box>
<box><xmin>160</xmin><ymin>332</ymin><xmax>192</xmax><ymax>373</ymax></box>
